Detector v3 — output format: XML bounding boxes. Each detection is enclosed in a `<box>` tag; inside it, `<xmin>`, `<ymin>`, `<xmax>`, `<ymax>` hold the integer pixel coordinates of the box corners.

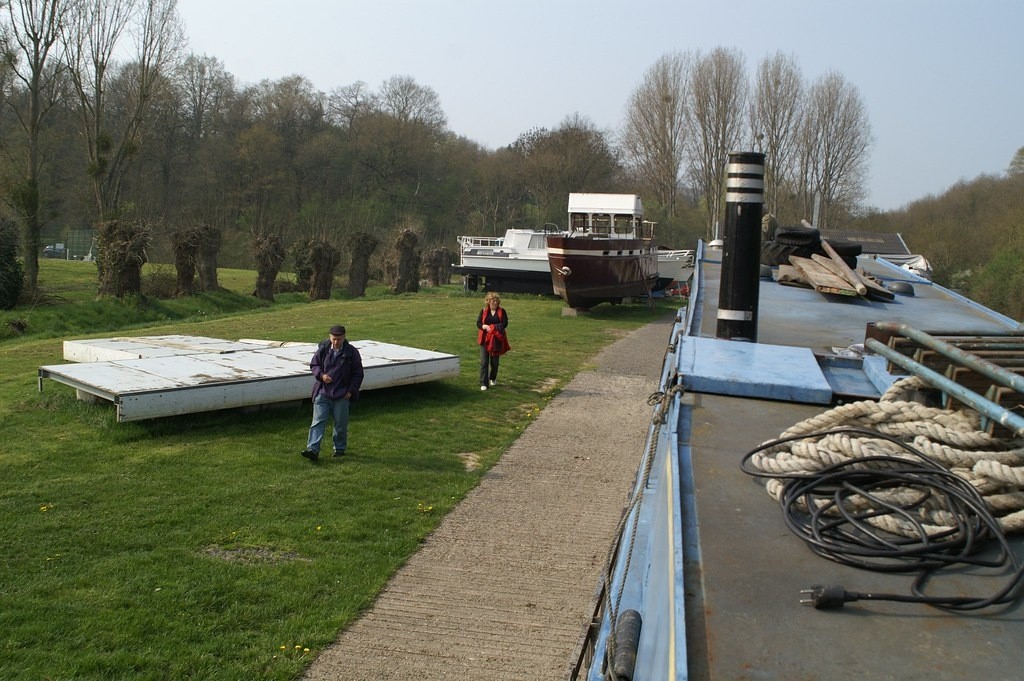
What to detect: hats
<box><xmin>329</xmin><ymin>326</ymin><xmax>345</xmax><ymax>336</ymax></box>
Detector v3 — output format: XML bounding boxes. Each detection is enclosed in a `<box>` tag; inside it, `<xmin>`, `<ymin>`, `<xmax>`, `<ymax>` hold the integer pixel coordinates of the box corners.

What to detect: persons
<box><xmin>300</xmin><ymin>325</ymin><xmax>366</xmax><ymax>464</ymax></box>
<box><xmin>473</xmin><ymin>290</ymin><xmax>510</xmax><ymax>392</ymax></box>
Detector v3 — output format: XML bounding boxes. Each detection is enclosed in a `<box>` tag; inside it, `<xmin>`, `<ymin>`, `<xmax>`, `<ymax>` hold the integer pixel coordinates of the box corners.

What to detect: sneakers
<box><xmin>301</xmin><ymin>449</ymin><xmax>318</xmax><ymax>460</ymax></box>
<box><xmin>331</xmin><ymin>449</ymin><xmax>344</xmax><ymax>458</ymax></box>
<box><xmin>481</xmin><ymin>385</ymin><xmax>487</xmax><ymax>391</ymax></box>
<box><xmin>490</xmin><ymin>379</ymin><xmax>496</xmax><ymax>386</ymax></box>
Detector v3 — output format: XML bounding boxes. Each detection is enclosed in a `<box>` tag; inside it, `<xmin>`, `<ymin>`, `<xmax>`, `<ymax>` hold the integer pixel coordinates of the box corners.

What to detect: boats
<box><xmin>635</xmin><ymin>256</ymin><xmax>695</xmax><ymax>298</ymax></box>
<box><xmin>546</xmin><ymin>192</ymin><xmax>660</xmax><ymax>309</ymax></box>
<box><xmin>450</xmin><ymin>222</ymin><xmax>695</xmax><ymax>295</ymax></box>
<box><xmin>584</xmin><ymin>151</ymin><xmax>1024</xmax><ymax>681</ymax></box>
